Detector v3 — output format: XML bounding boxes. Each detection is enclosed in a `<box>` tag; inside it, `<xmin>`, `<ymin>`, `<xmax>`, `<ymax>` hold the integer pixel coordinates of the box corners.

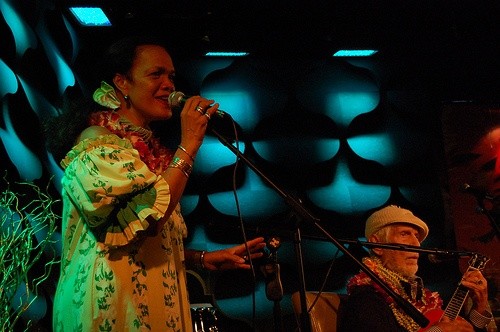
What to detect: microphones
<box><xmin>475</xmin><ymin>206</ymin><xmax>499</xmax><ymax>218</ymax></box>
<box><xmin>167</xmin><ymin>91</ymin><xmax>228</xmax><ymax>119</ymax></box>
<box><xmin>461</xmin><ymin>183</ymin><xmax>496</xmax><ymax>200</ymax></box>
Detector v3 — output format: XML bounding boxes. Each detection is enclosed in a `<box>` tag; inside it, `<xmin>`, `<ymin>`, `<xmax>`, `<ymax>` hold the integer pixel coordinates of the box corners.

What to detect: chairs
<box><xmin>292</xmin><ymin>290</ymin><xmax>348</xmax><ymax>332</ymax></box>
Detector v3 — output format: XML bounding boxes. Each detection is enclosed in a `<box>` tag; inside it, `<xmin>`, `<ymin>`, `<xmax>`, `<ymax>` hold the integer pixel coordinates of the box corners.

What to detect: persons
<box><xmin>344</xmin><ymin>204</ymin><xmax>487</xmax><ymax>332</ymax></box>
<box><xmin>44</xmin><ymin>37</ymin><xmax>267</xmax><ymax>332</ymax></box>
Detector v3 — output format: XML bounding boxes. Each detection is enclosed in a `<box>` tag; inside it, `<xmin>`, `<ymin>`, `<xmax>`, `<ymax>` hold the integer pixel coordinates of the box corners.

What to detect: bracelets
<box><xmin>167</xmin><ymin>156</ymin><xmax>192</xmax><ymax>177</ymax></box>
<box><xmin>243</xmin><ymin>255</ymin><xmax>247</xmax><ymax>260</ymax></box>
<box><xmin>200</xmin><ymin>251</ymin><xmax>206</xmax><ymax>263</ymax></box>
<box><xmin>178</xmin><ymin>145</ymin><xmax>195</xmax><ymax>162</ymax></box>
<box><xmin>426</xmin><ymin>326</ymin><xmax>442</xmax><ymax>332</ymax></box>
<box><xmin>469</xmin><ymin>308</ymin><xmax>493</xmax><ymax>329</ymax></box>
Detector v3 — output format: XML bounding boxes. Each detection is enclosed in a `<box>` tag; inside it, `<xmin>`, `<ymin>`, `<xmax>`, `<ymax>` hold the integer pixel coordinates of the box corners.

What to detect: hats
<box><xmin>365</xmin><ymin>205</ymin><xmax>429</xmax><ymax>242</ymax></box>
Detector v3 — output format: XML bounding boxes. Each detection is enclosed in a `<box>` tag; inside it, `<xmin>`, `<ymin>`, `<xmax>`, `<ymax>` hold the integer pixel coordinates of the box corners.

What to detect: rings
<box><xmin>196</xmin><ymin>105</ymin><xmax>205</xmax><ymax>115</ymax></box>
<box><xmin>475</xmin><ymin>279</ymin><xmax>482</xmax><ymax>285</ymax></box>
<box><xmin>205</xmin><ymin>112</ymin><xmax>211</xmax><ymax>119</ymax></box>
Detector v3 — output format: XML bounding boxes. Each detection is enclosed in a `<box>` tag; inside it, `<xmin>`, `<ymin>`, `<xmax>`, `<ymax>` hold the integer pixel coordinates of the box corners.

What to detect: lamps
<box><xmin>202</xmin><ymin>20</ymin><xmax>251</xmax><ymax>58</ymax></box>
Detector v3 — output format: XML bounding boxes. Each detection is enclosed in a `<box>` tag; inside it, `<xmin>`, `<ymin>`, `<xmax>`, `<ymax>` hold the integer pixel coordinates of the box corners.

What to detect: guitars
<box><xmin>416</xmin><ymin>251</ymin><xmax>491</xmax><ymax>332</ymax></box>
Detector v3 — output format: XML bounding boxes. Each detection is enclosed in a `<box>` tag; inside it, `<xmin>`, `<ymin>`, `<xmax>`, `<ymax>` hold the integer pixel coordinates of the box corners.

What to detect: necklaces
<box><xmin>346</xmin><ymin>257</ymin><xmax>443</xmax><ymax>318</ymax></box>
<box><xmin>90</xmin><ymin>111</ymin><xmax>172</xmax><ymax>175</ymax></box>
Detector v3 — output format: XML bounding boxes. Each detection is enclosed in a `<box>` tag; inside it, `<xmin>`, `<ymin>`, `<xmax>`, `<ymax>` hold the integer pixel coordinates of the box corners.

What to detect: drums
<box><xmin>190</xmin><ymin>301</ymin><xmax>219</xmax><ymax>332</ymax></box>
